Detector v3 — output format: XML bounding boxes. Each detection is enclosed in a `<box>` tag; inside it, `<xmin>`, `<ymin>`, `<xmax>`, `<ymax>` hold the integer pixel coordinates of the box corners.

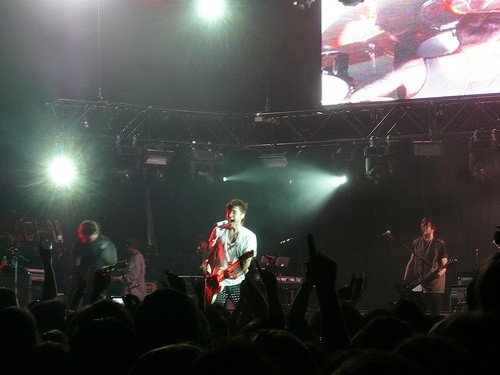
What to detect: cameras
<box><xmin>111</xmin><ymin>296</ymin><xmax>126</xmax><ymax>306</ymax></box>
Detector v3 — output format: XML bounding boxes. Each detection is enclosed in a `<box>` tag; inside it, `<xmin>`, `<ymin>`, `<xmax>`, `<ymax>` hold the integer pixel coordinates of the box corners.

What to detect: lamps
<box><xmin>467</xmin><ymin>130</ymin><xmax>500</xmax><ymax>183</ymax></box>
<box><xmin>143</xmin><ymin>148</ymin><xmax>173</xmax><ymax>166</ymax></box>
<box><xmin>363</xmin><ymin>136</ymin><xmax>394</xmax><ymax>183</ymax></box>
<box><xmin>411</xmin><ymin>141</ymin><xmax>442</xmax><ymax>158</ymax></box>
<box><xmin>265</xmin><ymin>156</ymin><xmax>287</xmax><ymax>167</ymax></box>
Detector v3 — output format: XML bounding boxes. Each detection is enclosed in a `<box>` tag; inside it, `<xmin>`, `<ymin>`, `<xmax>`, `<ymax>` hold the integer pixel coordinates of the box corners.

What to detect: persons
<box><xmin>0</xmin><ymin>233</ymin><xmax>500</xmax><ymax>375</ymax></box>
<box><xmin>403</xmin><ymin>216</ymin><xmax>449</xmax><ymax>315</ymax></box>
<box><xmin>70</xmin><ymin>220</ymin><xmax>117</xmax><ymax>304</ymax></box>
<box><xmin>202</xmin><ymin>199</ymin><xmax>258</xmax><ymax>307</ymax></box>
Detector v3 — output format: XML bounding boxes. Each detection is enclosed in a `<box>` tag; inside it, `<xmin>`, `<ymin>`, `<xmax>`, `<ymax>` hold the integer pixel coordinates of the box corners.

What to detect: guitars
<box><xmin>205</xmin><ymin>250</ymin><xmax>254</xmax><ymax>304</ymax></box>
<box><xmin>73</xmin><ymin>259</ymin><xmax>129</xmax><ymax>289</ymax></box>
<box><xmin>395</xmin><ymin>258</ymin><xmax>461</xmax><ymax>298</ymax></box>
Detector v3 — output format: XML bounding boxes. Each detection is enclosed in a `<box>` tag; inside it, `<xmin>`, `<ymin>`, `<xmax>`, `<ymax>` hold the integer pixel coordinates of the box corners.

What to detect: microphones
<box><xmin>383</xmin><ymin>230</ymin><xmax>391</xmax><ymax>236</ymax></box>
<box><xmin>280</xmin><ymin>238</ymin><xmax>294</xmax><ymax>245</ymax></box>
<box><xmin>215</xmin><ymin>220</ymin><xmax>231</xmax><ymax>227</ymax></box>
